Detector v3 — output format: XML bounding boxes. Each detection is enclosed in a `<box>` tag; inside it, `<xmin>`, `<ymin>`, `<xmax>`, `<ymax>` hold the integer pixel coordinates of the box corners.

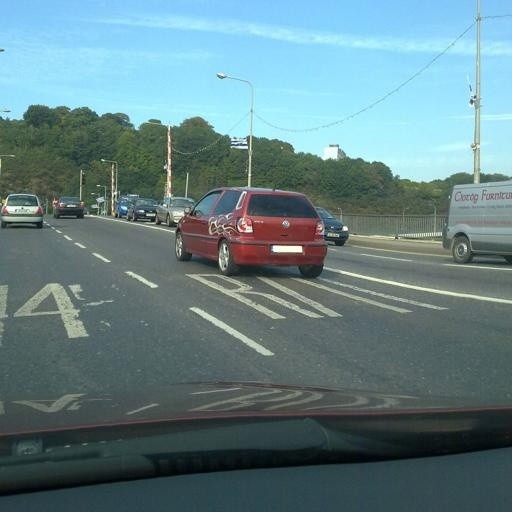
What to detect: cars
<box><xmin>53</xmin><ymin>197</ymin><xmax>86</xmax><ymax>218</ymax></box>
<box><xmin>174</xmin><ymin>183</ymin><xmax>328</xmax><ymax>278</ymax></box>
<box><xmin>114</xmin><ymin>196</ymin><xmax>199</xmax><ymax>227</ymax></box>
<box><xmin>0</xmin><ymin>194</ymin><xmax>44</xmax><ymax>229</ymax></box>
<box><xmin>314</xmin><ymin>207</ymin><xmax>349</xmax><ymax>246</ymax></box>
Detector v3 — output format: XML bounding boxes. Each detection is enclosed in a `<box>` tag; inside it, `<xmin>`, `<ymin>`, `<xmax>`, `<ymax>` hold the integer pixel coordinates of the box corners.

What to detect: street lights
<box><xmin>144</xmin><ymin>120</ymin><xmax>172</xmax><ymax>217</ymax></box>
<box><xmin>216</xmin><ymin>73</ymin><xmax>253</xmax><ymax>187</ymax></box>
<box><xmin>90</xmin><ymin>158</ymin><xmax>119</xmax><ymax>217</ymax></box>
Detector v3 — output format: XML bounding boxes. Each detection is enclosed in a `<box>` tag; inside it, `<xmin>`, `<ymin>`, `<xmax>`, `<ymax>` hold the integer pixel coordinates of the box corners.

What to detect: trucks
<box><xmin>442</xmin><ymin>180</ymin><xmax>512</xmax><ymax>263</ymax></box>
